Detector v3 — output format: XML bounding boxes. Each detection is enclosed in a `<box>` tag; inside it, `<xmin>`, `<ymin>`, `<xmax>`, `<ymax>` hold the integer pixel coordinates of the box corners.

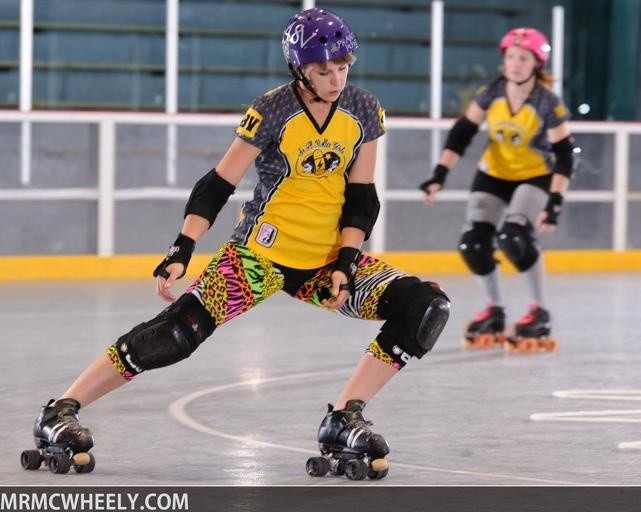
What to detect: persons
<box><xmin>34</xmin><ymin>8</ymin><xmax>450</xmax><ymax>455</ymax></box>
<box><xmin>423</xmin><ymin>29</ymin><xmax>575</xmax><ymax>338</ymax></box>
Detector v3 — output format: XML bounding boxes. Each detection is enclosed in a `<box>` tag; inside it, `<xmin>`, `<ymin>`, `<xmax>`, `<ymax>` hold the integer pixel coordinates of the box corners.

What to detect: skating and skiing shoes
<box><xmin>21</xmin><ymin>398</ymin><xmax>95</xmax><ymax>474</ymax></box>
<box><xmin>462</xmin><ymin>303</ymin><xmax>505</xmax><ymax>350</ymax></box>
<box><xmin>305</xmin><ymin>399</ymin><xmax>390</xmax><ymax>481</ymax></box>
<box><xmin>506</xmin><ymin>303</ymin><xmax>555</xmax><ymax>353</ymax></box>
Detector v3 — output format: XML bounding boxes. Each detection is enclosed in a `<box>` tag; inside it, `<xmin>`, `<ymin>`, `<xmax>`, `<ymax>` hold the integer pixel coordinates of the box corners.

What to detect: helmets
<box><xmin>281</xmin><ymin>7</ymin><xmax>359</xmax><ymax>80</ymax></box>
<box><xmin>498</xmin><ymin>27</ymin><xmax>551</xmax><ymax>70</ymax></box>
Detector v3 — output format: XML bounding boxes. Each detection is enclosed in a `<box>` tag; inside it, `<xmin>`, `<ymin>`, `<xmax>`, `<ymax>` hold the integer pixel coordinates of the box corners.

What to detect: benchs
<box><xmin>0</xmin><ymin>1</ymin><xmax>550</xmax><ymax>119</ymax></box>
